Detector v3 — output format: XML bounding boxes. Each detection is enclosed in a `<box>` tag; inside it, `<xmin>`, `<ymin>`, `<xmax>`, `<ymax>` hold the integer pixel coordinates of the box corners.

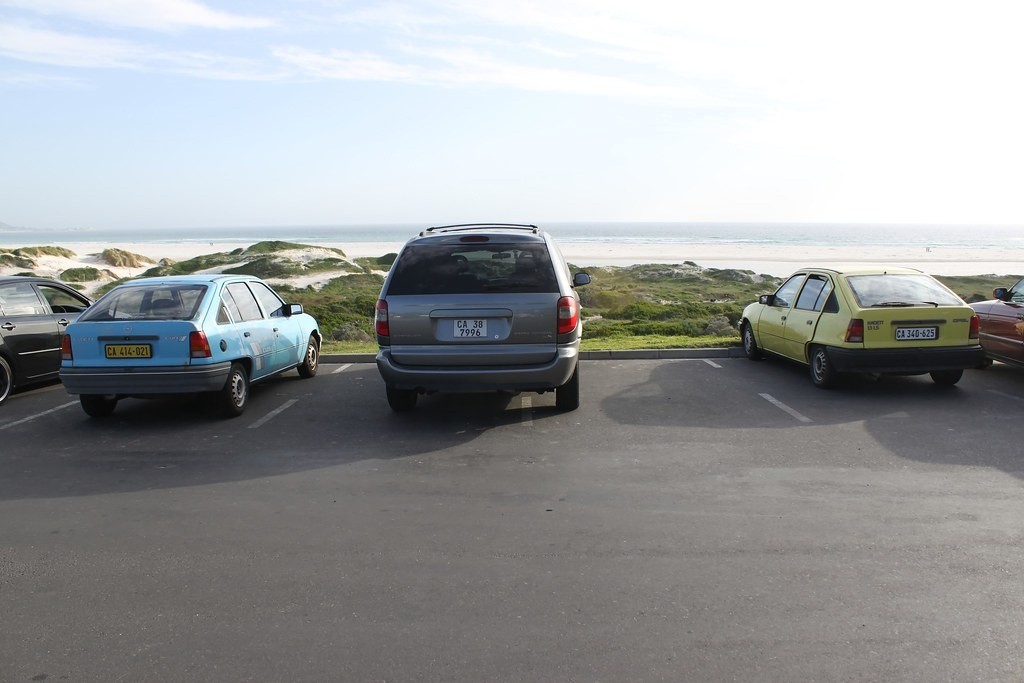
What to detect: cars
<box><xmin>0</xmin><ymin>274</ymin><xmax>135</xmax><ymax>406</ymax></box>
<box><xmin>734</xmin><ymin>265</ymin><xmax>988</xmax><ymax>392</ymax></box>
<box><xmin>966</xmin><ymin>275</ymin><xmax>1024</xmax><ymax>369</ymax></box>
<box><xmin>59</xmin><ymin>273</ymin><xmax>322</xmax><ymax>419</ymax></box>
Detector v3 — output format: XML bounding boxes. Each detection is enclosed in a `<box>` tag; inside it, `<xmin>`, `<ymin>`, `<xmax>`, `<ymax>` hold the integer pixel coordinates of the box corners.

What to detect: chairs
<box><xmin>429</xmin><ymin>254</ymin><xmax>477</xmax><ymax>292</ymax></box>
<box><xmin>152</xmin><ymin>299</ymin><xmax>182</xmax><ymax>319</ymax></box>
<box><xmin>507</xmin><ymin>250</ymin><xmax>543</xmax><ymax>288</ymax></box>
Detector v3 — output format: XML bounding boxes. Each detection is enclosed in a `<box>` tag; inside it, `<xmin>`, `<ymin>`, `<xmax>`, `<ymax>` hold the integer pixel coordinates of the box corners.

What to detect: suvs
<box><xmin>373</xmin><ymin>222</ymin><xmax>591</xmax><ymax>412</ymax></box>
